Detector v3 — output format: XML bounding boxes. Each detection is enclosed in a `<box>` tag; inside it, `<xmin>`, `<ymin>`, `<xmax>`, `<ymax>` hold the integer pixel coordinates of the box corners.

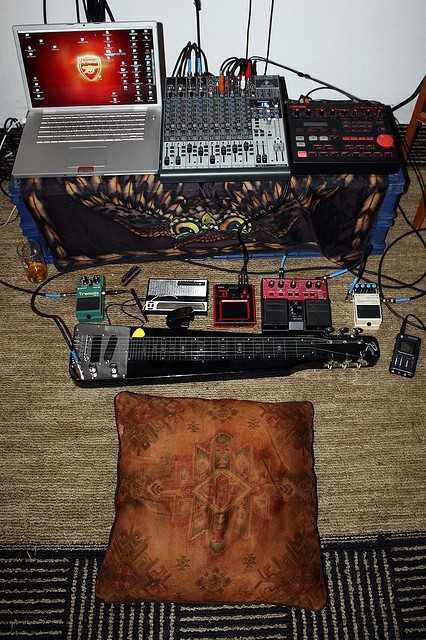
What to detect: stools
<box><xmin>404</xmin><ymin>75</ymin><xmax>426</xmax><ymax>233</ymax></box>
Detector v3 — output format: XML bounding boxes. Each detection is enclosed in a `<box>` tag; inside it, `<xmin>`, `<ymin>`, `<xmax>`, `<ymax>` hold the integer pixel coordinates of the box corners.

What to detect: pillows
<box><xmin>93</xmin><ymin>389</ymin><xmax>331</xmax><ymax>613</ymax></box>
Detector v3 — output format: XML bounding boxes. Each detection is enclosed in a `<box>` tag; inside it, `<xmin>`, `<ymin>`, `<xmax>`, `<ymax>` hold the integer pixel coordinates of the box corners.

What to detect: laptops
<box><xmin>11</xmin><ymin>20</ymin><xmax>164</xmax><ymax>179</ymax></box>
